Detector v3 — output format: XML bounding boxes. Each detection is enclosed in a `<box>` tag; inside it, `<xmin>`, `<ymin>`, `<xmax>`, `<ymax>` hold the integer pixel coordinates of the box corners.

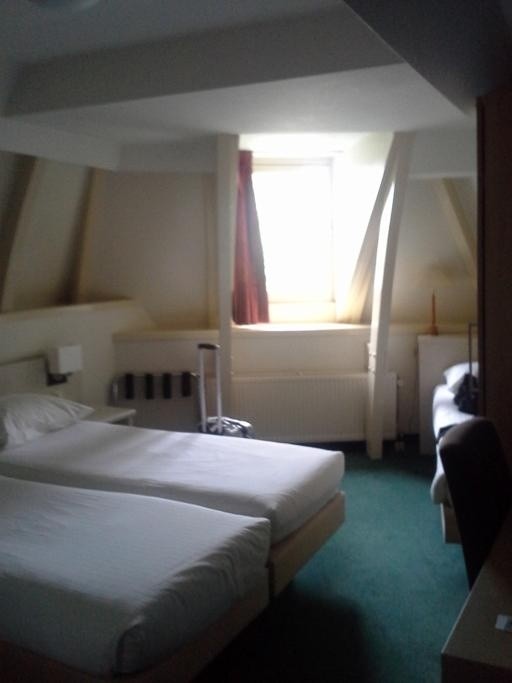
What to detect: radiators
<box><xmin>231</xmin><ymin>372</ymin><xmax>398</xmax><ymax>443</ymax></box>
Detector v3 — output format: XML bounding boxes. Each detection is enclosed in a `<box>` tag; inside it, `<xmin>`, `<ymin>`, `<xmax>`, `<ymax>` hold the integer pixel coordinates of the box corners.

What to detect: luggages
<box><xmin>195</xmin><ymin>343</ymin><xmax>253</xmax><ymax>438</ymax></box>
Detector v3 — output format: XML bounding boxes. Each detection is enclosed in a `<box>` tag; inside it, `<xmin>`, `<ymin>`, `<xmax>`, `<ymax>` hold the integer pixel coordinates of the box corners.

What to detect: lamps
<box><xmin>45</xmin><ymin>344</ymin><xmax>83</xmax><ymax>385</ymax></box>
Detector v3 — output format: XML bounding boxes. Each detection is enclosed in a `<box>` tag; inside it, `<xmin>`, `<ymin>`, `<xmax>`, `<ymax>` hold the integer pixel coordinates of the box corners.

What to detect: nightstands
<box><xmin>83</xmin><ymin>405</ymin><xmax>136</xmax><ymax>426</ymax></box>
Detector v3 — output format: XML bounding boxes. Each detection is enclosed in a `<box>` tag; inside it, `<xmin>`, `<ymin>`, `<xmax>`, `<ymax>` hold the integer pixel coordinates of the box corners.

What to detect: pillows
<box><xmin>442</xmin><ymin>361</ymin><xmax>479</xmax><ymax>392</ymax></box>
<box><xmin>0</xmin><ymin>393</ymin><xmax>94</xmax><ymax>451</ymax></box>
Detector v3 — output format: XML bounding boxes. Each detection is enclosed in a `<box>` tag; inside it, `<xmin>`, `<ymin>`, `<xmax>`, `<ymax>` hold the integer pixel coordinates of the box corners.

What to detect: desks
<box><xmin>441</xmin><ymin>526</ymin><xmax>512</xmax><ymax>683</ymax></box>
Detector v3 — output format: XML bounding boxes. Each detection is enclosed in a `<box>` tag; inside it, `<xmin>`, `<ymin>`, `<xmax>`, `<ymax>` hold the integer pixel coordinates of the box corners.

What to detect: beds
<box><xmin>432</xmin><ymin>383</ymin><xmax>481</xmax><ymax>544</ymax></box>
<box><xmin>0</xmin><ymin>419</ymin><xmax>348</xmax><ymax>602</ymax></box>
<box><xmin>0</xmin><ymin>474</ymin><xmax>273</xmax><ymax>683</ymax></box>
